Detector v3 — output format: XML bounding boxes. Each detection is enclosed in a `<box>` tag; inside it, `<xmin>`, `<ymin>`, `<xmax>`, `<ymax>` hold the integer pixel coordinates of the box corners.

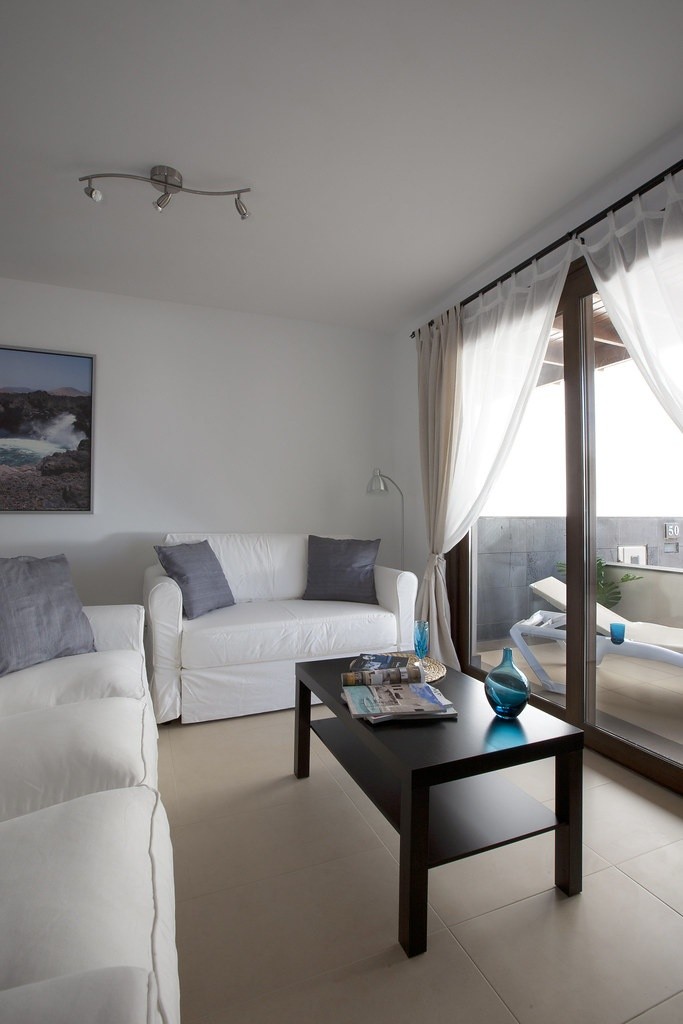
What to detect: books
<box><xmin>340</xmin><ymin>653</ymin><xmax>460</xmax><ymax>725</ymax></box>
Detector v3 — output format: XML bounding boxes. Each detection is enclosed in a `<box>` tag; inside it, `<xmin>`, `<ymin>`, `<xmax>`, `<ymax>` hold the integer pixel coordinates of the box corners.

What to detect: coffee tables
<box><xmin>291</xmin><ymin>648</ymin><xmax>582</xmax><ymax>958</ymax></box>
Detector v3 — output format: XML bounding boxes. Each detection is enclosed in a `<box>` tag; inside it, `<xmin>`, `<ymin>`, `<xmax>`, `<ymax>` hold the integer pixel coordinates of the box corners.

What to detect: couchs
<box><xmin>0</xmin><ymin>533</ymin><xmax>420</xmax><ymax>1023</ymax></box>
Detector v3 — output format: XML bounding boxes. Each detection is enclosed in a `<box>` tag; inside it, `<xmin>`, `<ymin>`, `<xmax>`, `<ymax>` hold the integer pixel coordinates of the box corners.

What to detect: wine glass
<box><xmin>414</xmin><ymin>620</ymin><xmax>429</xmax><ymax>676</ymax></box>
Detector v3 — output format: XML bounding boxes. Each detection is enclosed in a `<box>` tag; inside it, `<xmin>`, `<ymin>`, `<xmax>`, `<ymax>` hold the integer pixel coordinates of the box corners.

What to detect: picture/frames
<box><xmin>0</xmin><ymin>345</ymin><xmax>97</xmax><ymax>515</ymax></box>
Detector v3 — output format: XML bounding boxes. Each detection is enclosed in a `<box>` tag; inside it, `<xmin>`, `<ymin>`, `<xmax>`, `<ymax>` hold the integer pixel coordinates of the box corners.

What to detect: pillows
<box><xmin>153</xmin><ymin>539</ymin><xmax>235</xmax><ymax>620</ymax></box>
<box><xmin>303</xmin><ymin>535</ymin><xmax>382</xmax><ymax>604</ymax></box>
<box><xmin>0</xmin><ymin>554</ymin><xmax>97</xmax><ymax>676</ymax></box>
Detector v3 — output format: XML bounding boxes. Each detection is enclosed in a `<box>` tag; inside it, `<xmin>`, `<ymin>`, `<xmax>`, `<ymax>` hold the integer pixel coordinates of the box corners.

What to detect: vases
<box><xmin>484</xmin><ymin>648</ymin><xmax>527</xmax><ymax>719</ymax></box>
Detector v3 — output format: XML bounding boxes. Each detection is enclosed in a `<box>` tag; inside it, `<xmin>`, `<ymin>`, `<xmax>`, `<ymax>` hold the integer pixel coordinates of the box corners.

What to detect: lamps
<box><xmin>76</xmin><ymin>166</ymin><xmax>250</xmax><ymax>221</ymax></box>
<box><xmin>365</xmin><ymin>469</ymin><xmax>404</xmax><ymax>570</ymax></box>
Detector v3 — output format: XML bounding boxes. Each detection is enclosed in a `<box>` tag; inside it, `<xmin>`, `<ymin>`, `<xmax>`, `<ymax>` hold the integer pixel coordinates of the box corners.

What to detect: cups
<box><xmin>610</xmin><ymin>623</ymin><xmax>626</xmax><ymax>645</ymax></box>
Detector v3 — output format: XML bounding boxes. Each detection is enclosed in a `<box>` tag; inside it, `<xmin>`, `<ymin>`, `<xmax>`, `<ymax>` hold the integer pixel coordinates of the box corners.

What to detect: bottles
<box><xmin>484</xmin><ymin>648</ymin><xmax>531</xmax><ymax>719</ymax></box>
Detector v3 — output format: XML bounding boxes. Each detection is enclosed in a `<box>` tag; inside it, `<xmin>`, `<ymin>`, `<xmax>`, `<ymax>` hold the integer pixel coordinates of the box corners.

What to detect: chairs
<box><xmin>509</xmin><ymin>577</ymin><xmax>683</xmax><ymax>693</ymax></box>
